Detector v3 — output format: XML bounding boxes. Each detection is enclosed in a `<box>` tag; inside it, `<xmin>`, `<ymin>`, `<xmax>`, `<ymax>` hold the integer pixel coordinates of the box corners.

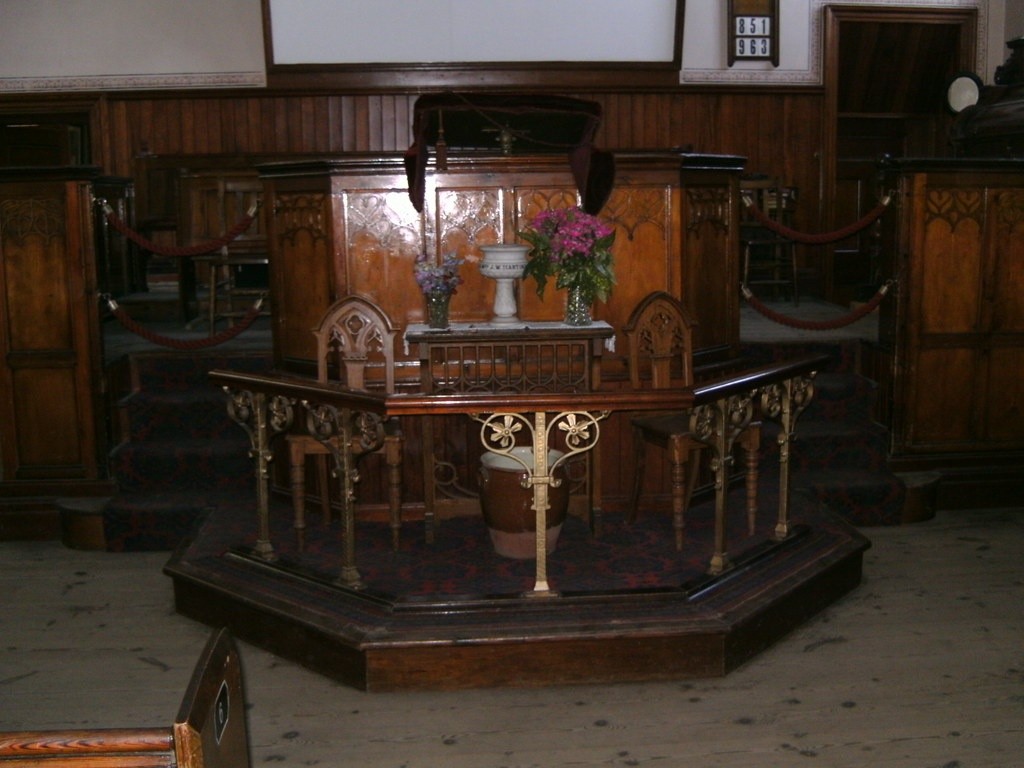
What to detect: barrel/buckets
<box><xmin>481</xmin><ymin>447</ymin><xmax>570</xmax><ymax>561</ymax></box>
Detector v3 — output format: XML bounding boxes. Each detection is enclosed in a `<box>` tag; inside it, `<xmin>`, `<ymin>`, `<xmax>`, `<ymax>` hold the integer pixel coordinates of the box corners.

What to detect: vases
<box><xmin>564</xmin><ymin>284</ymin><xmax>592</xmax><ymax>328</ymax></box>
<box><xmin>426</xmin><ymin>291</ymin><xmax>451</xmax><ymax>328</ymax></box>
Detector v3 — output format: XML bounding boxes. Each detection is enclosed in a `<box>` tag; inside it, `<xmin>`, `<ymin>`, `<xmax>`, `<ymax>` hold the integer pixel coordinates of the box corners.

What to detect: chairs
<box><xmin>740</xmin><ymin>174</ymin><xmax>799</xmax><ymax>307</ymax></box>
<box><xmin>285</xmin><ymin>293</ymin><xmax>408</xmax><ymax>550</ymax></box>
<box><xmin>191</xmin><ymin>168</ymin><xmax>274</xmax><ymax>339</ymax></box>
<box><xmin>621</xmin><ymin>291</ymin><xmax>765</xmax><ymax>552</ymax></box>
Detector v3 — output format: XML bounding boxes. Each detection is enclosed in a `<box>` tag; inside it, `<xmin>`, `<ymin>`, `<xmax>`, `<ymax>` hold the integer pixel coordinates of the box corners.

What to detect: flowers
<box><xmin>413</xmin><ymin>251</ymin><xmax>464</xmax><ymax>299</ymax></box>
<box><xmin>515</xmin><ymin>204</ymin><xmax>617</xmax><ymax>306</ymax></box>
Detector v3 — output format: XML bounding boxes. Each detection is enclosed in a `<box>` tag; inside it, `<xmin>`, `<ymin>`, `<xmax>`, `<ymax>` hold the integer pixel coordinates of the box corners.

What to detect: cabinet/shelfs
<box><xmin>0</xmin><ymin>167</ymin><xmax>135</xmax><ymax>550</ymax></box>
<box><xmin>878</xmin><ymin>154</ymin><xmax>1024</xmax><ymax>474</ymax></box>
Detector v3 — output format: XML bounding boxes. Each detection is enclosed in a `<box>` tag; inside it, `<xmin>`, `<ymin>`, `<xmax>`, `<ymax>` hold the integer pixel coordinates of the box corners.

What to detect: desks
<box><xmin>402</xmin><ymin>318</ymin><xmax>617</xmax><ymax>541</ymax></box>
